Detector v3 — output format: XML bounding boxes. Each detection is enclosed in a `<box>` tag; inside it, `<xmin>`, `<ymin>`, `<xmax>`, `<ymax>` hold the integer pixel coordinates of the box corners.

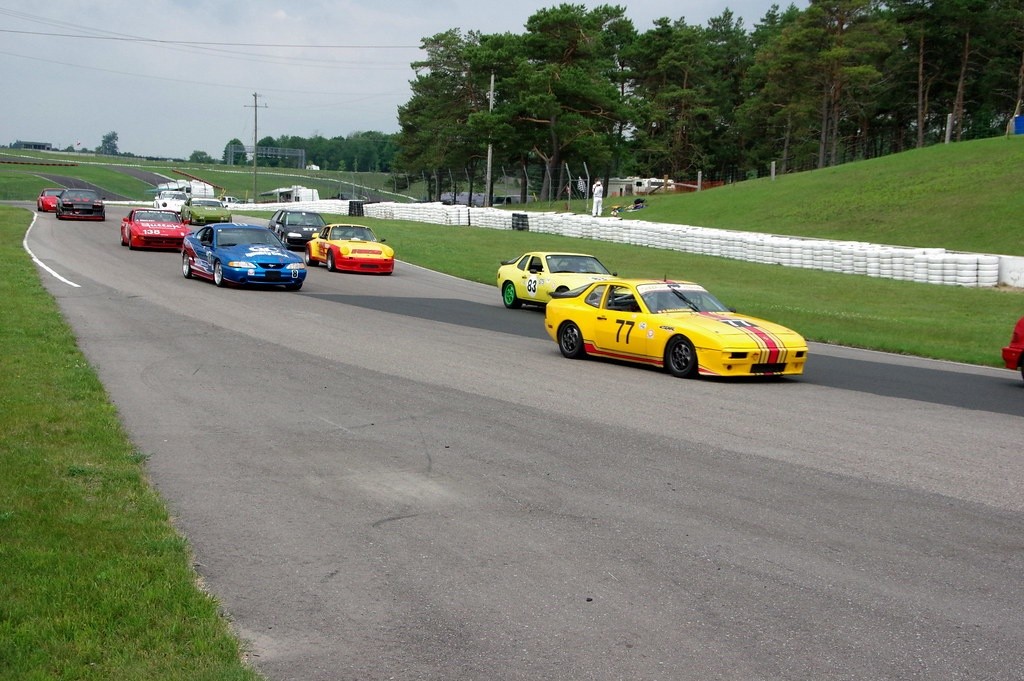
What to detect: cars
<box><xmin>182</xmin><ymin>223</ymin><xmax>307</xmax><ymax>292</ymax></box>
<box><xmin>268</xmin><ymin>210</ymin><xmax>329</xmax><ymax>251</ymax></box>
<box><xmin>305</xmin><ymin>223</ymin><xmax>394</xmax><ymax>276</ymax></box>
<box><xmin>38</xmin><ymin>188</ymin><xmax>66</xmax><ymax>211</ymax></box>
<box><xmin>153</xmin><ymin>191</ymin><xmax>190</xmax><ymax>213</ymax></box>
<box><xmin>121</xmin><ymin>208</ymin><xmax>192</xmax><ymax>250</ymax></box>
<box><xmin>498</xmin><ymin>252</ymin><xmax>620</xmax><ymax>310</ymax></box>
<box><xmin>180</xmin><ymin>196</ymin><xmax>233</xmax><ymax>226</ymax></box>
<box><xmin>55</xmin><ymin>189</ymin><xmax>107</xmax><ymax>221</ymax></box>
<box><xmin>544</xmin><ymin>276</ymin><xmax>807</xmax><ymax>380</ymax></box>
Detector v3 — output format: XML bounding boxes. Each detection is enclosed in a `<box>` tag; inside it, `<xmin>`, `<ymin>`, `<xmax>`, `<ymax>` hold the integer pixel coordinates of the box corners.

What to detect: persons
<box><xmin>577</xmin><ymin>261</ymin><xmax>588</xmax><ymax>273</ymax></box>
<box><xmin>592</xmin><ymin>178</ymin><xmax>602</xmax><ymax>217</ymax></box>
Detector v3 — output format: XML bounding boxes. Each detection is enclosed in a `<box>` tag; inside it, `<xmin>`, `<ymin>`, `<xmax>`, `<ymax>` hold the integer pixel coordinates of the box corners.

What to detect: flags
<box><xmin>77</xmin><ymin>143</ymin><xmax>79</xmax><ymax>146</ymax></box>
<box><xmin>577</xmin><ymin>176</ymin><xmax>585</xmax><ymax>193</ymax></box>
<box><xmin>566</xmin><ymin>187</ymin><xmax>569</xmax><ymax>193</ymax></box>
